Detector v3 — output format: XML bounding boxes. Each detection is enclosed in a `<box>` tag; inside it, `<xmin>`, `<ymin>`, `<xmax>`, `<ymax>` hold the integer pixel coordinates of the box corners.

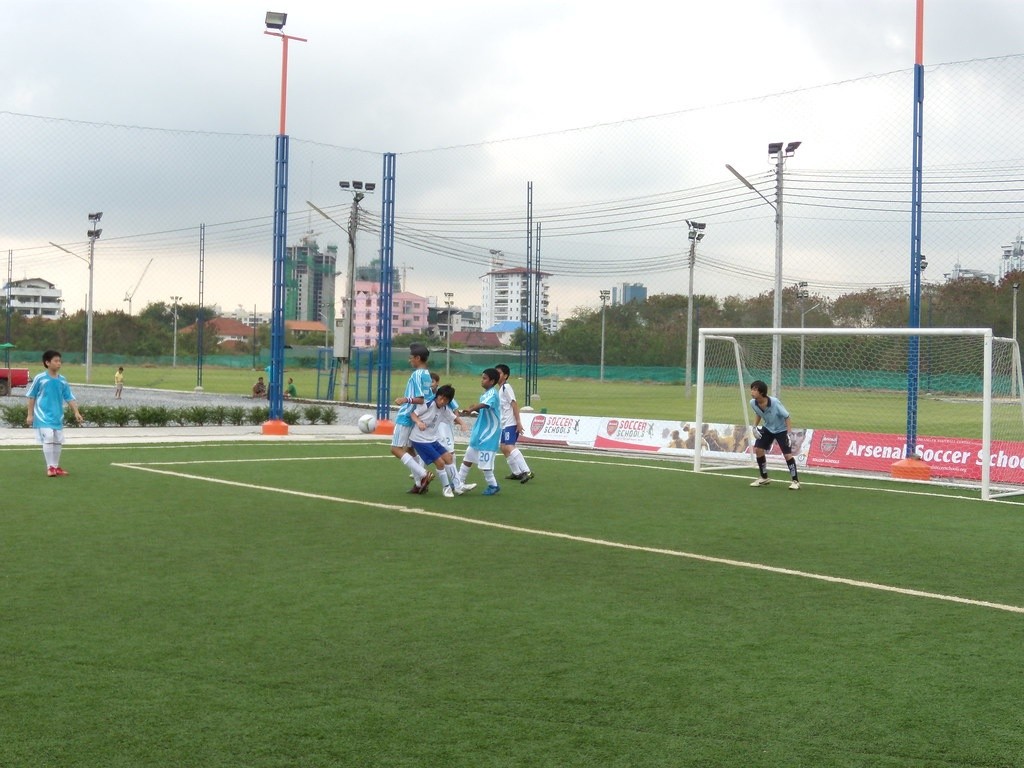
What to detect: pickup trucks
<box><xmin>0</xmin><ymin>367</ymin><xmax>31</xmax><ymax>396</ymax></box>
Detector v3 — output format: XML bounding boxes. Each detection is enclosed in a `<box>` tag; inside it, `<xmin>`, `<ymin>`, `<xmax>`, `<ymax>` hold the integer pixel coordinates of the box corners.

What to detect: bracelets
<box><xmin>406</xmin><ymin>397</ymin><xmax>413</xmax><ymax>404</ymax></box>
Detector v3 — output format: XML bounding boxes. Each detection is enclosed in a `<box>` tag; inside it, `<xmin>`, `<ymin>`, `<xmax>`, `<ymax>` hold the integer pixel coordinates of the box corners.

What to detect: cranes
<box><xmin>393</xmin><ymin>262</ymin><xmax>414</xmax><ymax>293</ymax></box>
<box><xmin>122</xmin><ymin>258</ymin><xmax>154</xmax><ymax>315</ymax></box>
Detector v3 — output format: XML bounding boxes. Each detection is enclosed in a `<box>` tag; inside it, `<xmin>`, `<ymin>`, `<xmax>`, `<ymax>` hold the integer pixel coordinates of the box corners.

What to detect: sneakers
<box><xmin>454</xmin><ymin>483</ymin><xmax>476</xmax><ymax>495</ymax></box>
<box><xmin>504</xmin><ymin>472</ymin><xmax>523</xmax><ymax>480</ymax></box>
<box><xmin>442</xmin><ymin>486</ymin><xmax>455</xmax><ymax>498</ymax></box>
<box><xmin>481</xmin><ymin>484</ymin><xmax>500</xmax><ymax>496</ymax></box>
<box><xmin>750</xmin><ymin>475</ymin><xmax>770</xmax><ymax>486</ymax></box>
<box><xmin>789</xmin><ymin>480</ymin><xmax>800</xmax><ymax>489</ymax></box>
<box><xmin>520</xmin><ymin>471</ymin><xmax>534</xmax><ymax>484</ymax></box>
<box><xmin>406</xmin><ymin>484</ymin><xmax>428</xmax><ymax>494</ymax></box>
<box><xmin>47</xmin><ymin>466</ymin><xmax>69</xmax><ymax>477</ymax></box>
<box><xmin>418</xmin><ymin>470</ymin><xmax>434</xmax><ymax>494</ymax></box>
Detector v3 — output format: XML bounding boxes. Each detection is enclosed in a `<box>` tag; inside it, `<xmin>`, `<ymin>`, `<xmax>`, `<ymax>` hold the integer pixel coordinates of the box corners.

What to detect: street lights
<box><xmin>725</xmin><ymin>140</ymin><xmax>802</xmax><ymax>397</ymax></box>
<box><xmin>684</xmin><ymin>219</ymin><xmax>707</xmax><ymax>394</ymax></box>
<box><xmin>50</xmin><ymin>210</ymin><xmax>103</xmax><ymax>382</ymax></box>
<box><xmin>1012</xmin><ymin>283</ymin><xmax>1021</xmax><ymax>397</ymax></box>
<box><xmin>599</xmin><ymin>290</ymin><xmax>611</xmax><ymax>382</ymax></box>
<box><xmin>443</xmin><ymin>292</ymin><xmax>454</xmax><ymax>375</ymax></box>
<box><xmin>309</xmin><ymin>179</ymin><xmax>376</xmax><ymax>403</ymax></box>
<box><xmin>170</xmin><ymin>295</ymin><xmax>182</xmax><ymax>366</ymax></box>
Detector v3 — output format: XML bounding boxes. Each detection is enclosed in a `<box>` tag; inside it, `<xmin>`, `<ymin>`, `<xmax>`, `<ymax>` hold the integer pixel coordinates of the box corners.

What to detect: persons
<box><xmin>264</xmin><ymin>366</ymin><xmax>271</xmax><ymax>401</ymax></box>
<box><xmin>449</xmin><ymin>368</ymin><xmax>501</xmax><ymax>495</ymax></box>
<box><xmin>26</xmin><ymin>349</ymin><xmax>83</xmax><ymax>477</ymax></box>
<box><xmin>390</xmin><ymin>344</ymin><xmax>477</xmax><ymax>497</ymax></box>
<box><xmin>669</xmin><ymin>424</ymin><xmax>806</xmax><ymax>457</ymax></box>
<box><xmin>495</xmin><ymin>364</ymin><xmax>535</xmax><ymax>484</ymax></box>
<box><xmin>253</xmin><ymin>377</ymin><xmax>268</xmax><ymax>400</ymax></box>
<box><xmin>283</xmin><ymin>378</ymin><xmax>298</xmax><ymax>397</ymax></box>
<box><xmin>114</xmin><ymin>367</ymin><xmax>124</xmax><ymax>399</ymax></box>
<box><xmin>749</xmin><ymin>380</ymin><xmax>800</xmax><ymax>490</ymax></box>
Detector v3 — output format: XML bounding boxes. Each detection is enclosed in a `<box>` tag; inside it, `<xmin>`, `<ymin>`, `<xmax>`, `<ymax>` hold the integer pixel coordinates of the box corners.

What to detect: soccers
<box><xmin>357</xmin><ymin>413</ymin><xmax>378</xmax><ymax>432</ymax></box>
